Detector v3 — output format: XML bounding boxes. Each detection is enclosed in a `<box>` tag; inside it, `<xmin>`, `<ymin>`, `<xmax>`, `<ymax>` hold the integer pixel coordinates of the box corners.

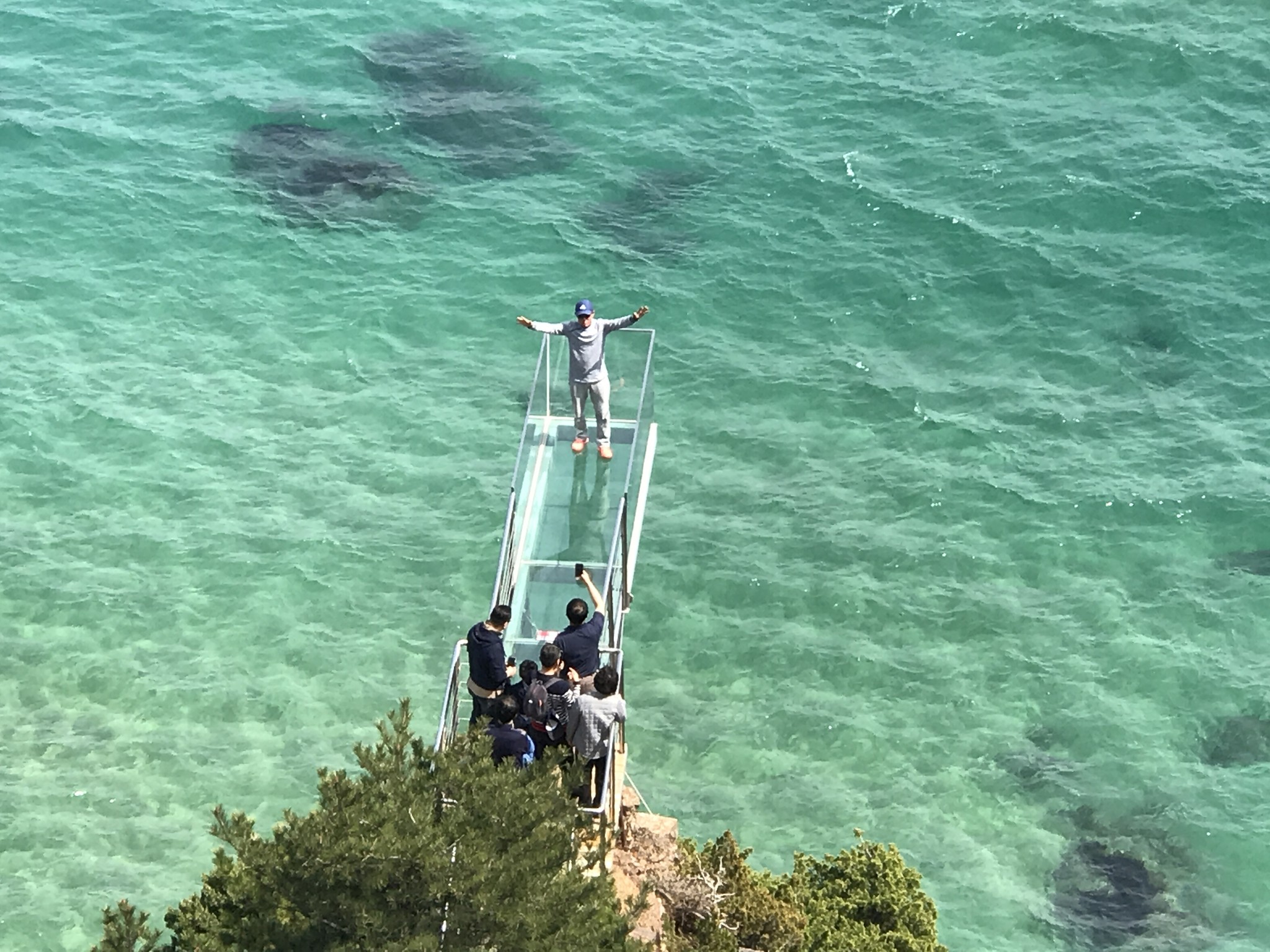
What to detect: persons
<box><xmin>565</xmin><ymin>663</ymin><xmax>628</xmax><ymax>818</ymax></box>
<box><xmin>553</xmin><ymin>569</ymin><xmax>606</xmax><ymax>691</ymax></box>
<box><xmin>467</xmin><ymin>605</ymin><xmax>517</xmax><ymax>729</ymax></box>
<box><xmin>515</xmin><ymin>297</ymin><xmax>650</xmax><ymax>459</ymax></box>
<box><xmin>466</xmin><ymin>569</ymin><xmax>628</xmax><ymax>818</ymax></box>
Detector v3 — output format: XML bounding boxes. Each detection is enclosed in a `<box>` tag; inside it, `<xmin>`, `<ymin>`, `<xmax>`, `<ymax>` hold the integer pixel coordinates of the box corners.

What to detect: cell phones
<box><xmin>575</xmin><ymin>564</ymin><xmax>583</xmax><ymax>577</ymax></box>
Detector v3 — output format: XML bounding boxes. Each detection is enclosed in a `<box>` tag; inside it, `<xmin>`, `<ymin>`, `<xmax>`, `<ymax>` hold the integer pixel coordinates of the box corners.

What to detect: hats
<box><xmin>575</xmin><ymin>299</ymin><xmax>594</xmax><ymax>317</ymax></box>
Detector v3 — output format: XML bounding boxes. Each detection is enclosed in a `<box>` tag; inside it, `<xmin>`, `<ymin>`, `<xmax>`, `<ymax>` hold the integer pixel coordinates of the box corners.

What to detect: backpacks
<box><xmin>522</xmin><ymin>668</ymin><xmax>565</xmax><ymax>725</ymax></box>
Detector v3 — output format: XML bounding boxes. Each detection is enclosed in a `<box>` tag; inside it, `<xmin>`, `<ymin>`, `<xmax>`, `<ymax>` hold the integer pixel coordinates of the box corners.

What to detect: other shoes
<box><xmin>597</xmin><ymin>443</ymin><xmax>612</xmax><ymax>459</ymax></box>
<box><xmin>571</xmin><ymin>437</ymin><xmax>589</xmax><ymax>451</ymax></box>
